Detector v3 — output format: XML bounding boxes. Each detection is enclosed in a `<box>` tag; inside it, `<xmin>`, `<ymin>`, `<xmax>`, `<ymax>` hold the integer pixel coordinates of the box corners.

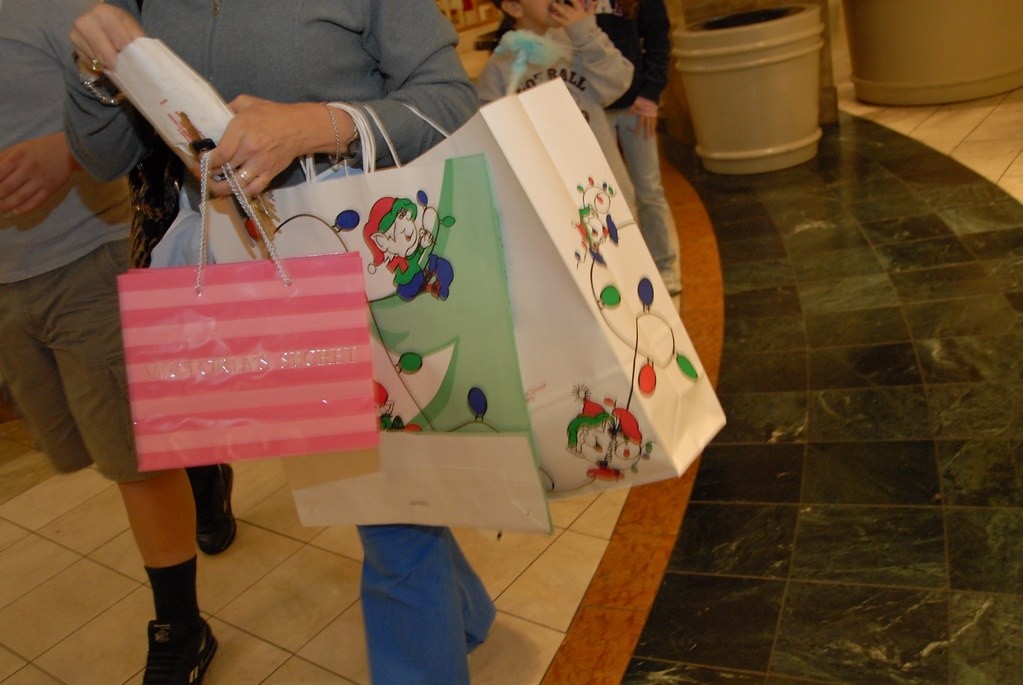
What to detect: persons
<box><xmin>71</xmin><ymin>0</ymin><xmax>495</xmax><ymax>685</ymax></box>
<box><xmin>0</xmin><ymin>0</ymin><xmax>237</xmax><ymax>685</ymax></box>
<box><xmin>478</xmin><ymin>1</ymin><xmax>683</xmax><ymax>316</ymax></box>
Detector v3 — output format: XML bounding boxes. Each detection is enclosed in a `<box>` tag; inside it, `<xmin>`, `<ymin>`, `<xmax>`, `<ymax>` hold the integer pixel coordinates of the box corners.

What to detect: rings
<box><xmin>257</xmin><ymin>176</ymin><xmax>268</xmax><ymax>190</ymax></box>
<box><xmin>91</xmin><ymin>58</ymin><xmax>99</xmax><ymax>70</ymax></box>
<box><xmin>236</xmin><ymin>168</ymin><xmax>251</xmax><ymax>184</ymax></box>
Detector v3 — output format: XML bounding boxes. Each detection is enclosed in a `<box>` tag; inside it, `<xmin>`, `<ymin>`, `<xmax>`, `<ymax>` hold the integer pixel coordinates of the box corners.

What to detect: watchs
<box><xmin>340</xmin><ymin>126</ymin><xmax>360</xmax><ymax>160</ymax></box>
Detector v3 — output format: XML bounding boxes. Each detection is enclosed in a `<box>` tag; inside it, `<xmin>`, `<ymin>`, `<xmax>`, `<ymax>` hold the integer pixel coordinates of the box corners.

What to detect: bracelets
<box><xmin>75</xmin><ymin>71</ymin><xmax>126</xmax><ymax>107</ymax></box>
<box><xmin>319</xmin><ymin>101</ymin><xmax>340</xmax><ymax>165</ymax></box>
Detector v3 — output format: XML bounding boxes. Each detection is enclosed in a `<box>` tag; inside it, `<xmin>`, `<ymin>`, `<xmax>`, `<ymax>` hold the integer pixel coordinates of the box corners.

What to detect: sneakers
<box><xmin>142</xmin><ymin>613</ymin><xmax>218</xmax><ymax>684</ymax></box>
<box><xmin>189</xmin><ymin>464</ymin><xmax>237</xmax><ymax>554</ymax></box>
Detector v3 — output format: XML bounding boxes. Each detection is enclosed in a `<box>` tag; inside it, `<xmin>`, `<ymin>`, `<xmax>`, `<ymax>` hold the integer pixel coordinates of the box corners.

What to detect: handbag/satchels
<box><xmin>115</xmin><ymin>70</ymin><xmax>728</xmax><ymax>535</ymax></box>
